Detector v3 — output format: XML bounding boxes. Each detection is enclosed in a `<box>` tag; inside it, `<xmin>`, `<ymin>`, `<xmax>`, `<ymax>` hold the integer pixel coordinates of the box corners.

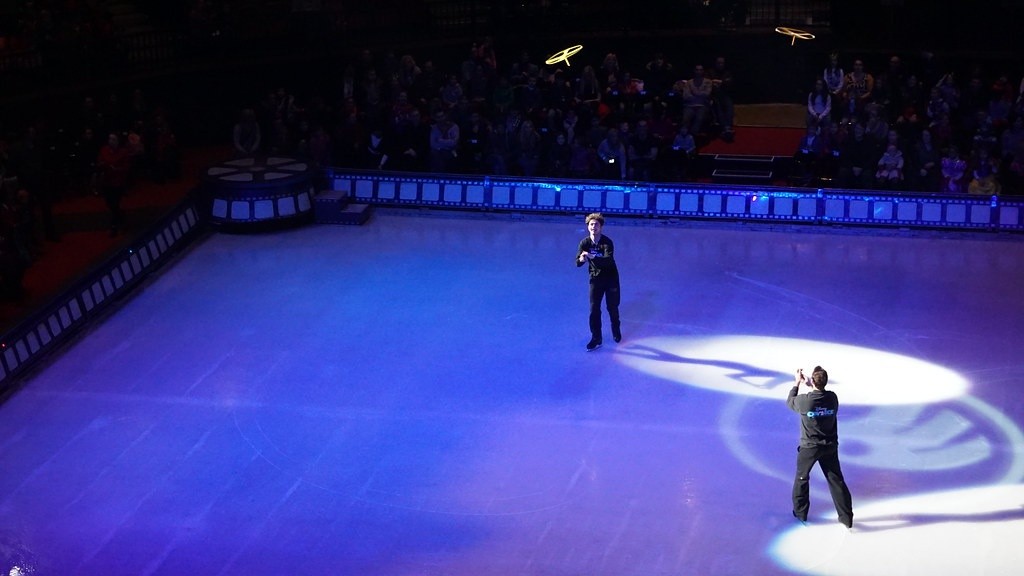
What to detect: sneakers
<box><xmin>791</xmin><ymin>510</ymin><xmax>808</xmax><ymax>526</ymax></box>
<box><xmin>838</xmin><ymin>515</ymin><xmax>853</xmax><ymax>531</ymax></box>
<box><xmin>612</xmin><ymin>328</ymin><xmax>621</xmax><ymax>344</ymax></box>
<box><xmin>586</xmin><ymin>336</ymin><xmax>603</xmax><ymax>352</ymax></box>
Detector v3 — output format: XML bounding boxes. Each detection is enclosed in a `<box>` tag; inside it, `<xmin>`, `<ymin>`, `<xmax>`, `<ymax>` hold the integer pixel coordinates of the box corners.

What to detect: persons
<box><xmin>231</xmin><ymin>32</ymin><xmax>1024</xmax><ymax>196</ymax></box>
<box><xmin>575</xmin><ymin>212</ymin><xmax>621</xmax><ymax>351</ymax></box>
<box><xmin>786</xmin><ymin>365</ymin><xmax>854</xmax><ymax>528</ymax></box>
<box><xmin>2</xmin><ymin>91</ymin><xmax>183</xmax><ymax>304</ymax></box>
